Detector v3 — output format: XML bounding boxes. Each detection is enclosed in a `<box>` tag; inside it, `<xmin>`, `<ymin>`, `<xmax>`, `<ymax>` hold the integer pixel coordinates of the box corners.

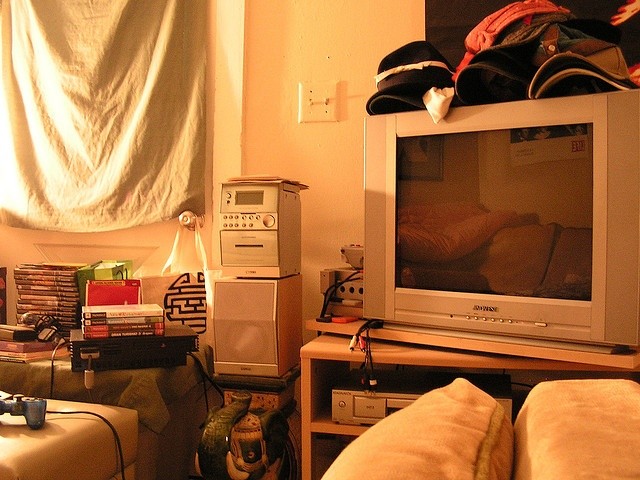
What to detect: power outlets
<box><xmin>298</xmin><ymin>81</ymin><xmax>341</xmax><ymax>123</ymax></box>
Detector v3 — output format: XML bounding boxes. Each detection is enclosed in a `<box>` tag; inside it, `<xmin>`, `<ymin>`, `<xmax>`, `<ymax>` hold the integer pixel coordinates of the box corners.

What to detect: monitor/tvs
<box><xmin>363</xmin><ymin>89</ymin><xmax>640</xmax><ymax>356</ymax></box>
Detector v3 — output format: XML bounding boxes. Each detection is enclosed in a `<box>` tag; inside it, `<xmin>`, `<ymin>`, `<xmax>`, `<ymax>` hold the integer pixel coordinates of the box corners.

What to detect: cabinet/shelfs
<box><xmin>300</xmin><ymin>333</ymin><xmax>639</xmax><ymax>480</ymax></box>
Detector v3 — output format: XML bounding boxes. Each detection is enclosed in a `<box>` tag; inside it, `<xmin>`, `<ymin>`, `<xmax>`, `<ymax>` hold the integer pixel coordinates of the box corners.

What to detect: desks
<box><xmin>0</xmin><ymin>344</ymin><xmax>225</xmax><ymax>479</ymax></box>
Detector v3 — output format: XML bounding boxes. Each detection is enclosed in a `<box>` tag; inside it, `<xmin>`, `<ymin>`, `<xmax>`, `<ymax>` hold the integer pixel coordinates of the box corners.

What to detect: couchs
<box><xmin>513</xmin><ymin>379</ymin><xmax>638</xmax><ymax>480</ymax></box>
<box><xmin>0</xmin><ymin>394</ymin><xmax>139</xmax><ymax>480</ymax></box>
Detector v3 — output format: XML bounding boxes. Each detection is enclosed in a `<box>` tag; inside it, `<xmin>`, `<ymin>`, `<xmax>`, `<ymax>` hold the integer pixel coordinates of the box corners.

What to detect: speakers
<box><xmin>213</xmin><ymin>275</ymin><xmax>303</xmax><ymax>377</ymax></box>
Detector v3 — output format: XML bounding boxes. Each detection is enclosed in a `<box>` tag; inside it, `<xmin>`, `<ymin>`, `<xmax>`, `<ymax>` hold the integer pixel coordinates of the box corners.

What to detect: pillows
<box><xmin>534</xmin><ymin>228</ymin><xmax>592</xmax><ymax>297</ymax></box>
<box><xmin>320</xmin><ymin>378</ymin><xmax>516</xmax><ymax>480</ymax></box>
<box><xmin>473</xmin><ymin>221</ymin><xmax>561</xmax><ymax>296</ymax></box>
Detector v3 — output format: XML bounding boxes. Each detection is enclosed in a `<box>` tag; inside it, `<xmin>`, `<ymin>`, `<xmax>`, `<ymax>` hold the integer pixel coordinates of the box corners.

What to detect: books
<box><xmin>13</xmin><ymin>263</ymin><xmax>80</xmax><ymax>341</ymax></box>
<box><xmin>81</xmin><ymin>303</ymin><xmax>165</xmax><ymax>337</ymax></box>
<box><xmin>0</xmin><ymin>325</ymin><xmax>68</xmax><ymax>364</ymax></box>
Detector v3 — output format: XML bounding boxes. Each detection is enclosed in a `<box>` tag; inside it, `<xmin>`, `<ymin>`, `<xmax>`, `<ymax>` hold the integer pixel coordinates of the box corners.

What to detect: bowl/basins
<box><xmin>340</xmin><ymin>247</ymin><xmax>363</xmax><ymax>270</ymax></box>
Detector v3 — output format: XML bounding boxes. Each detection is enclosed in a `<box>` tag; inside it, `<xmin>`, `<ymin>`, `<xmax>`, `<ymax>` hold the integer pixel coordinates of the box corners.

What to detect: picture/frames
<box><xmin>396</xmin><ymin>136</ymin><xmax>445</xmax><ymax>181</ymax></box>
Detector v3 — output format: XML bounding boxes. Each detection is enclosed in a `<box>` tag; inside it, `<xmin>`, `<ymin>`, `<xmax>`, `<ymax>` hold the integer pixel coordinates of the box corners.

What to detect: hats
<box><xmin>366</xmin><ymin>40</ymin><xmax>457</xmax><ymax>115</ymax></box>
<box><xmin>452</xmin><ymin>0</ymin><xmax>639</xmax><ymax>105</ymax></box>
